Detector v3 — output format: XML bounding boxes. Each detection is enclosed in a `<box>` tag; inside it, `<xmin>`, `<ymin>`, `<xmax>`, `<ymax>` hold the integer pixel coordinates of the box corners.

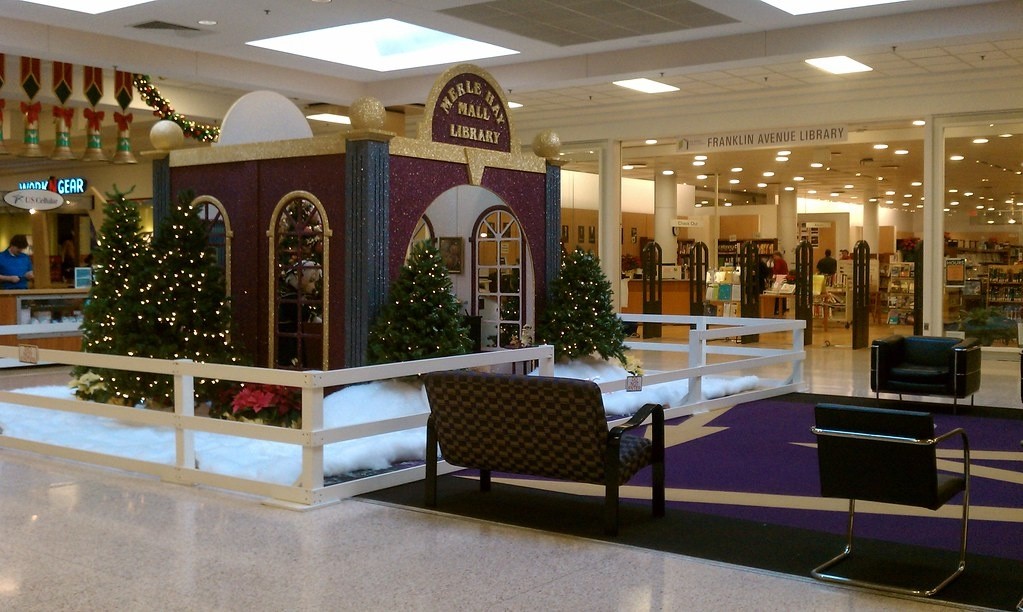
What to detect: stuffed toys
<box><xmin>278</xmin><ymin>260</ymin><xmax>323</xmax><ymax>367</ymax></box>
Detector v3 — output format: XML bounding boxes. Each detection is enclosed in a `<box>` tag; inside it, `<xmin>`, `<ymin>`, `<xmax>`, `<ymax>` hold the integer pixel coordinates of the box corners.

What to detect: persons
<box><xmin>0</xmin><ymin>233</ymin><xmax>35</xmax><ymax>289</ymax></box>
<box><xmin>771</xmin><ymin>252</ymin><xmax>789</xmax><ymax>315</ymax></box>
<box><xmin>742</xmin><ymin>248</ymin><xmax>769</xmax><ymax>308</ymax></box>
<box><xmin>838</xmin><ymin>249</ymin><xmax>852</xmax><ymax>261</ymax></box>
<box><xmin>61</xmin><ymin>231</ymin><xmax>77</xmax><ymax>281</ymax></box>
<box><xmin>816</xmin><ymin>249</ymin><xmax>837</xmax><ymax>287</ymax></box>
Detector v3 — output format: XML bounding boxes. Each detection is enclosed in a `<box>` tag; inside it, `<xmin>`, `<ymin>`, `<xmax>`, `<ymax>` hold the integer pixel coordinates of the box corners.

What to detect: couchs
<box><xmin>870</xmin><ymin>335</ymin><xmax>981</xmax><ymax>412</ymax></box>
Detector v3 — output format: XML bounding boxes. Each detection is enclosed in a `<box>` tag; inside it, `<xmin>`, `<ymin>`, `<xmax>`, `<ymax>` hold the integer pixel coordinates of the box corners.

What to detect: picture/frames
<box><xmin>562</xmin><ymin>225</ymin><xmax>569</xmax><ymax>243</ymax></box>
<box><xmin>486</xmin><ymin>222</ymin><xmax>496</xmax><ymax>242</ymax></box>
<box><xmin>589</xmin><ymin>226</ymin><xmax>595</xmax><ymax>243</ymax></box>
<box><xmin>630</xmin><ymin>227</ymin><xmax>638</xmax><ymax>244</ymax></box>
<box><xmin>578</xmin><ymin>225</ymin><xmax>585</xmax><ymax>243</ymax></box>
<box><xmin>503</xmin><ymin>222</ymin><xmax>512</xmax><ymax>241</ymax></box>
<box><xmin>440</xmin><ymin>237</ymin><xmax>464</xmax><ymax>272</ymax></box>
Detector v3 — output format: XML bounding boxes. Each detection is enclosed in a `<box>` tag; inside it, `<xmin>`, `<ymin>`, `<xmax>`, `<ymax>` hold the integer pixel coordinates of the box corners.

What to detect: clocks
<box><xmin>673</xmin><ymin>227</ymin><xmax>681</xmax><ymax>236</ymax></box>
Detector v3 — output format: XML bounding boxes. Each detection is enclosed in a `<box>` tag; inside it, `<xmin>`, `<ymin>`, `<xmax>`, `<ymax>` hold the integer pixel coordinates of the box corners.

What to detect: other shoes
<box><xmin>782</xmin><ymin>312</ymin><xmax>785</xmax><ymax>315</ymax></box>
<box><xmin>774</xmin><ymin>311</ymin><xmax>779</xmax><ymax>315</ymax></box>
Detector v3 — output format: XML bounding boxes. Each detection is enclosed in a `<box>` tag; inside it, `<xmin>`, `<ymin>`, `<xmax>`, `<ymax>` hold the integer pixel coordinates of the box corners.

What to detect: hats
<box><xmin>840</xmin><ymin>249</ymin><xmax>849</xmax><ymax>254</ymax></box>
<box><xmin>11</xmin><ymin>234</ymin><xmax>28</xmax><ymax>249</ymax></box>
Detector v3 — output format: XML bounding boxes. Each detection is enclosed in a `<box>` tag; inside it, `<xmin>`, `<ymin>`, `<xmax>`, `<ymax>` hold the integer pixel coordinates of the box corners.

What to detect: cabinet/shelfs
<box><xmin>955</xmin><ymin>247</ymin><xmax>1010</xmax><ymax>301</ymax></box>
<box><xmin>987</xmin><ymin>265</ymin><xmax>1023</xmax><ymax>319</ymax></box>
<box><xmin>456</xmin><ymin>315</ymin><xmax>484</xmax><ymax>354</ymax></box>
<box><xmin>886</xmin><ymin>258</ymin><xmax>966</xmax><ymax>325</ymax></box>
<box><xmin>647</xmin><ymin>238</ymin><xmax>778</xmax><ymax>279</ymax></box>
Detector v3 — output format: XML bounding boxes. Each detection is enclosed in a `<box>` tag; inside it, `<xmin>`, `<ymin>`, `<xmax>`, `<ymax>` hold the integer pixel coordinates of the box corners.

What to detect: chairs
<box><xmin>809</xmin><ymin>403</ymin><xmax>971</xmax><ymax>597</ymax></box>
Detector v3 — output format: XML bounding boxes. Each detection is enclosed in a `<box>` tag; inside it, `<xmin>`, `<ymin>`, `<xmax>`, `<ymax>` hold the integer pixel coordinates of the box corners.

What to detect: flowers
<box><xmin>901</xmin><ymin>232</ymin><xmax>921</xmax><ymax>262</ymax></box>
<box><xmin>987</xmin><ymin>234</ymin><xmax>1001</xmax><ymax>245</ymax></box>
<box><xmin>220</xmin><ymin>385</ymin><xmax>301</xmax><ymax>427</ymax></box>
<box><xmin>618</xmin><ymin>354</ymin><xmax>646</xmax><ymax>377</ymax></box>
<box><xmin>621</xmin><ymin>253</ymin><xmax>639</xmax><ymax>272</ymax></box>
<box><xmin>69</xmin><ymin>374</ymin><xmax>132</xmax><ymax>405</ymax></box>
<box><xmin>944</xmin><ymin>232</ymin><xmax>951</xmax><ymax>243</ymax></box>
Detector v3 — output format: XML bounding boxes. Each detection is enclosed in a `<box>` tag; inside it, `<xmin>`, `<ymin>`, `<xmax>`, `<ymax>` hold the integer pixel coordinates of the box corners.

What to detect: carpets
<box><xmin>324</xmin><ymin>390</ymin><xmax>1023</xmax><ymax>612</ymax></box>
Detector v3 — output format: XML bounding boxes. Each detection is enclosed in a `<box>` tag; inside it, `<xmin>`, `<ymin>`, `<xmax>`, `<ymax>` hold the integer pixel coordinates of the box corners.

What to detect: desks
<box><xmin>759</xmin><ymin>294</ymin><xmax>830</xmax><ymax>333</ymax></box>
<box><xmin>620</xmin><ymin>279</ymin><xmax>706</xmax><ymax>316</ymax></box>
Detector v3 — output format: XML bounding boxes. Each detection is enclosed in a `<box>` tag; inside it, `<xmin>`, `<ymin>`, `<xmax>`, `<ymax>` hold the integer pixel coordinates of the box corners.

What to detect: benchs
<box><xmin>420</xmin><ymin>371</ymin><xmax>667</xmax><ymax>534</ymax></box>
